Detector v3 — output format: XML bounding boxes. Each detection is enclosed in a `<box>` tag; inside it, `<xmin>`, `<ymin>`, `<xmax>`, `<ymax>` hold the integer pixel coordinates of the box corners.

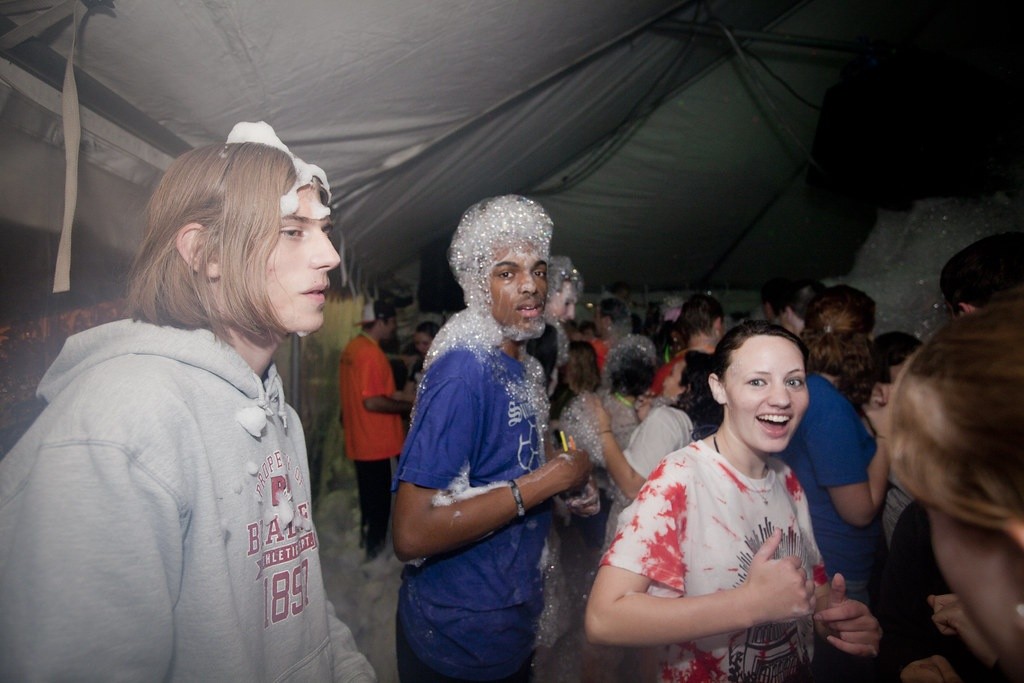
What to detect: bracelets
<box><xmin>599</xmin><ymin>430</ymin><xmax>612</xmax><ymax>435</ymax></box>
<box><xmin>509</xmin><ymin>480</ymin><xmax>525</xmax><ymax>516</ymax></box>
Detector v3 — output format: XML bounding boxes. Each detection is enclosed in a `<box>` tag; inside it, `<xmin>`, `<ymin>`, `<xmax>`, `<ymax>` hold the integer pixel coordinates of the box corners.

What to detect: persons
<box><xmin>869</xmin><ymin>331</ymin><xmax>923</xmax><ymax>545</ymax></box>
<box><xmin>340</xmin><ymin>300</ymin><xmax>414</xmax><ymax>561</ymax></box>
<box><xmin>775</xmin><ymin>285</ymin><xmax>892</xmax><ymax>683</ymax></box>
<box><xmin>0</xmin><ymin>143</ymin><xmax>379</xmax><ymax>682</ymax></box>
<box><xmin>391</xmin><ymin>194</ymin><xmax>601</xmax><ymax>683</ymax></box>
<box><xmin>888</xmin><ymin>290</ymin><xmax>1024</xmax><ymax>683</ymax></box>
<box><xmin>382</xmin><ymin>321</ymin><xmax>439</xmax><ymax>400</ymax></box>
<box><xmin>889</xmin><ymin>231</ymin><xmax>1024</xmax><ymax>683</ymax></box>
<box><xmin>584</xmin><ymin>320</ymin><xmax>883</xmax><ymax>683</ymax></box>
<box><xmin>525</xmin><ymin>274</ymin><xmax>789</xmax><ymax>557</ymax></box>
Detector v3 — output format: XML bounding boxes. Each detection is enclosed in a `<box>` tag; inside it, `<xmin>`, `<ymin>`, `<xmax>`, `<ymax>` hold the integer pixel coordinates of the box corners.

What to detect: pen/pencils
<box><xmin>569</xmin><ymin>435</ymin><xmax>576</xmax><ymax>450</ymax></box>
<box><xmin>560</xmin><ymin>431</ymin><xmax>568</xmax><ymax>452</ymax></box>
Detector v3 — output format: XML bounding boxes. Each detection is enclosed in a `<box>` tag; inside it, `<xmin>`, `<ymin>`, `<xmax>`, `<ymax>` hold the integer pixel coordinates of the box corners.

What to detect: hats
<box><xmin>354</xmin><ymin>299</ymin><xmax>396</xmax><ymax>327</ymax></box>
